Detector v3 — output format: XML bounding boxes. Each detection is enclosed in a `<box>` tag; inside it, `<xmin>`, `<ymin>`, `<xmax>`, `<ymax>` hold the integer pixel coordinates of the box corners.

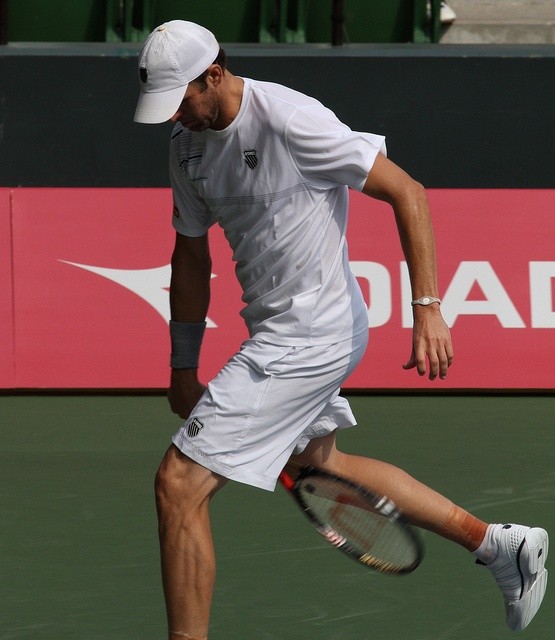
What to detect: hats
<box><xmin>134</xmin><ymin>20</ymin><xmax>219</xmax><ymax>125</ymax></box>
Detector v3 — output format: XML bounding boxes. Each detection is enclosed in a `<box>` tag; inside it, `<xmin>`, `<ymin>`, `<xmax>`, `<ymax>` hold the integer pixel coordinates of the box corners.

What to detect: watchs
<box><xmin>411</xmin><ymin>295</ymin><xmax>442</xmax><ymax>306</ymax></box>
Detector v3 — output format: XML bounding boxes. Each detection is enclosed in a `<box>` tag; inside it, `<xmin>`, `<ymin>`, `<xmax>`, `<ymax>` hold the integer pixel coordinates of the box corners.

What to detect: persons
<box><xmin>133</xmin><ymin>20</ymin><xmax>549</xmax><ymax>640</ymax></box>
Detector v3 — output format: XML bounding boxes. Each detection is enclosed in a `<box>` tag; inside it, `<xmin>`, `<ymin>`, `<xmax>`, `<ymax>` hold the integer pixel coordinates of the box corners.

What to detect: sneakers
<box><xmin>475</xmin><ymin>524</ymin><xmax>549</xmax><ymax>632</ymax></box>
